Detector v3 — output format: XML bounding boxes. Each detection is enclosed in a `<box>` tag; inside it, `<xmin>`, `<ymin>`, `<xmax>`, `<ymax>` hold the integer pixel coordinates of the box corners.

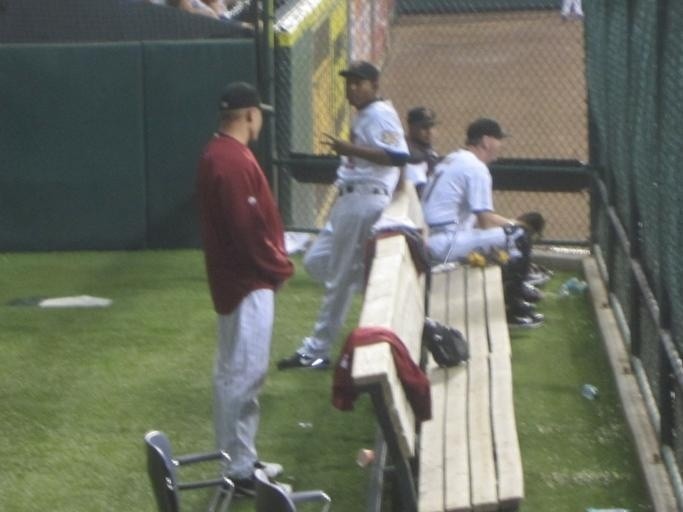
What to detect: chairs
<box><xmin>141</xmin><ymin>430</ymin><xmax>331</xmax><ymax>511</ymax></box>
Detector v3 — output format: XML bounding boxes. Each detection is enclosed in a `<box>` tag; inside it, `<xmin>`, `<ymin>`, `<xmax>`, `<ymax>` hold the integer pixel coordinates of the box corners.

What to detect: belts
<box><xmin>339</xmin><ymin>183</ymin><xmax>388</xmax><ymax>198</ymax></box>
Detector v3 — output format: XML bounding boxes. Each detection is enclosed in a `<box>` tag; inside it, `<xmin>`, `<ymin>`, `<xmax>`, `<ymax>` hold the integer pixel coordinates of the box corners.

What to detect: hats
<box><xmin>466</xmin><ymin>116</ymin><xmax>512</xmax><ymax>139</ymax></box>
<box><xmin>521</xmin><ymin>210</ymin><xmax>545</xmax><ymax>238</ymax></box>
<box><xmin>406</xmin><ymin>105</ymin><xmax>441</xmax><ymax>126</ymax></box>
<box><xmin>337</xmin><ymin>60</ymin><xmax>380</xmax><ymax>80</ymax></box>
<box><xmin>217</xmin><ymin>80</ymin><xmax>275</xmax><ymax>112</ymax></box>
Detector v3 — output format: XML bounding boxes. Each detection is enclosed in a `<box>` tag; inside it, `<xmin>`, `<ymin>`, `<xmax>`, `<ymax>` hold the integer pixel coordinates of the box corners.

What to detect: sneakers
<box><xmin>276</xmin><ymin>352</ymin><xmax>331</xmax><ymax>370</ymax></box>
<box><xmin>508</xmin><ymin>261</ymin><xmax>555</xmax><ymax>328</ymax></box>
<box><xmin>220</xmin><ymin>460</ymin><xmax>294</xmax><ymax>497</ymax></box>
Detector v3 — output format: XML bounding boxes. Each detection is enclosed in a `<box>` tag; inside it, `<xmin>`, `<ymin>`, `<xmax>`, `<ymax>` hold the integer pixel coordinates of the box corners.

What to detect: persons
<box><xmin>277</xmin><ymin>61</ymin><xmax>411</xmax><ymax>369</ymax></box>
<box><xmin>419</xmin><ymin>119</ymin><xmax>545</xmax><ymax>328</ymax></box>
<box><xmin>196</xmin><ymin>83</ymin><xmax>293</xmax><ymax>490</ymax></box>
<box><xmin>405</xmin><ymin>108</ymin><xmax>553</xmax><ymax>298</ymax></box>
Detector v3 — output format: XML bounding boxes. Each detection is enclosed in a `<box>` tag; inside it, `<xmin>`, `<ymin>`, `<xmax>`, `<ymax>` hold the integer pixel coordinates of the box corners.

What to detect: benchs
<box><xmin>347</xmin><ymin>186</ymin><xmax>523</xmax><ymax>512</ymax></box>
